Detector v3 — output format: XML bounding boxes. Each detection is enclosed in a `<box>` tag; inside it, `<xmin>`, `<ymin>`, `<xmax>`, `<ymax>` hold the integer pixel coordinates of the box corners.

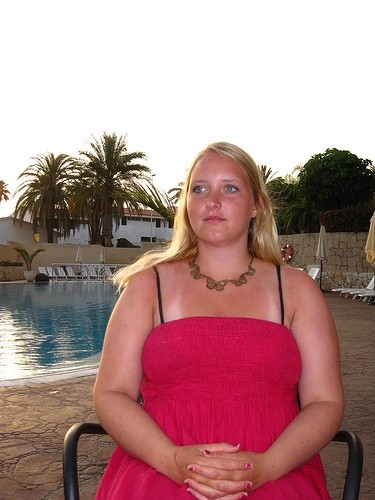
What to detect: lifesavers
<box><xmin>281</xmin><ymin>243</ymin><xmax>295</xmax><ymax>262</ymax></box>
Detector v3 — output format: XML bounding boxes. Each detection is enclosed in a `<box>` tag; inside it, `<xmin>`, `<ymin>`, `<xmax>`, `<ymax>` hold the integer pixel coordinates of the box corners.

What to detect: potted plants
<box><xmin>11</xmin><ymin>247</ymin><xmax>45</xmax><ymax>282</ymax></box>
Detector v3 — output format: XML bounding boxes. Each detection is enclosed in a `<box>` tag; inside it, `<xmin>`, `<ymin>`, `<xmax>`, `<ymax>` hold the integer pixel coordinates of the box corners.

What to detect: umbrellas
<box><xmin>316</xmin><ymin>223</ymin><xmax>329</xmax><ymax>293</ymax></box>
<box><xmin>364</xmin><ymin>208</ymin><xmax>375</xmax><ymax>290</ymax></box>
<box><xmin>98</xmin><ymin>244</ymin><xmax>106</xmax><ymax>264</ymax></box>
<box><xmin>75</xmin><ymin>244</ymin><xmax>83</xmax><ymax>274</ymax></box>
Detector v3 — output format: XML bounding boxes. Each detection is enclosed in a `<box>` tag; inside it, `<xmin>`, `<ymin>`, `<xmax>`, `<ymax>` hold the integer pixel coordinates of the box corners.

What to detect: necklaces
<box><xmin>187</xmin><ymin>250</ymin><xmax>257</xmax><ymax>293</ymax></box>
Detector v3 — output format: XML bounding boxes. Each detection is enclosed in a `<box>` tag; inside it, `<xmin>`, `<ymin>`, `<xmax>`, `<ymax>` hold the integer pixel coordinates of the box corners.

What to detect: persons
<box><xmin>93</xmin><ymin>141</ymin><xmax>346</xmax><ymax>500</ymax></box>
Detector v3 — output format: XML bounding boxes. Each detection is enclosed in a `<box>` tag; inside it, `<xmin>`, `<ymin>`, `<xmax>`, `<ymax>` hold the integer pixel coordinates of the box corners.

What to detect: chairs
<box><xmin>308</xmin><ymin>267</ymin><xmax>319</xmax><ymax>280</ymax></box>
<box><xmin>331</xmin><ymin>275</ymin><xmax>375</xmax><ymax>303</ymax></box>
<box><xmin>37</xmin><ymin>266</ymin><xmax>114</xmax><ymax>280</ymax></box>
<box><xmin>62</xmin><ymin>422</ymin><xmax>363</xmax><ymax>500</ymax></box>
